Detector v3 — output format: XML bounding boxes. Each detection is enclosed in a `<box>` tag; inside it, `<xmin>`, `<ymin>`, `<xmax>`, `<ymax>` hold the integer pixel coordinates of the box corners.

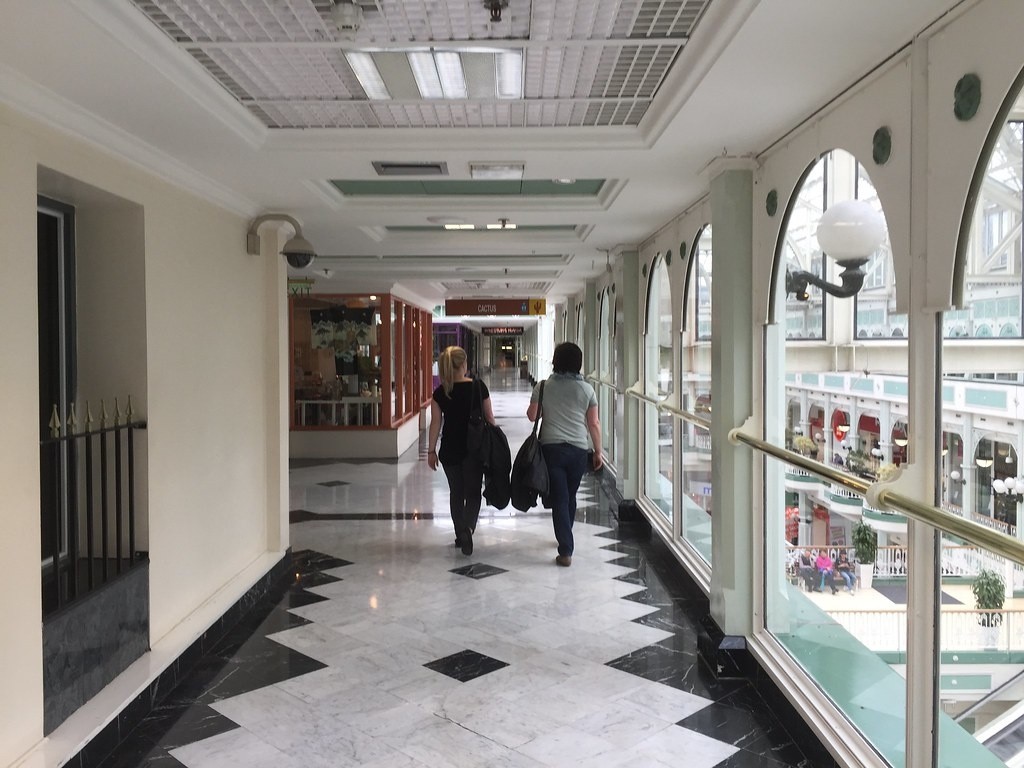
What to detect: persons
<box><xmin>833</xmin><ymin>549</ymin><xmax>857</xmax><ymax>594</ymax></box>
<box><xmin>525</xmin><ymin>341</ymin><xmax>604</xmax><ymax>567</ymax></box>
<box><xmin>798</xmin><ymin>549</ymin><xmax>823</xmax><ymax>593</ymax></box>
<box><xmin>426</xmin><ymin>346</ymin><xmax>494</xmax><ymax>557</ymax></box>
<box><xmin>834</xmin><ymin>453</ymin><xmax>843</xmax><ymax>465</ymax></box>
<box><xmin>816</xmin><ymin>549</ymin><xmax>839</xmax><ymax>596</ymax></box>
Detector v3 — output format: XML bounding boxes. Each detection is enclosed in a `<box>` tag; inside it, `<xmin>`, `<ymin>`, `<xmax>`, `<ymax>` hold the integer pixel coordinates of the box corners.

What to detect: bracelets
<box><xmin>428</xmin><ymin>451</ymin><xmax>435</xmax><ymax>455</ymax></box>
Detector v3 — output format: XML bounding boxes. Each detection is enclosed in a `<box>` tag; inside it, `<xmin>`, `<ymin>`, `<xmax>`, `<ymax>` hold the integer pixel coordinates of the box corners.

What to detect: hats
<box><xmin>840</xmin><ymin>550</ymin><xmax>846</xmax><ymax>554</ymax></box>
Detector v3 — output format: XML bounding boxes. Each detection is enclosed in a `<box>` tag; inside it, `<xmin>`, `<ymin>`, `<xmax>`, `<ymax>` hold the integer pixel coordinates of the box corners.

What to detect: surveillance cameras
<box><xmin>279</xmin><ymin>234</ymin><xmax>318</xmax><ymax>269</ymax></box>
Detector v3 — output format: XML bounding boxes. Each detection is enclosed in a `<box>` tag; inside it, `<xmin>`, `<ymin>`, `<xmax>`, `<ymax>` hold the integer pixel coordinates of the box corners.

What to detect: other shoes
<box><xmin>850</xmin><ymin>589</ymin><xmax>854</xmax><ymax>595</ymax></box>
<box><xmin>556</xmin><ymin>554</ymin><xmax>571</xmax><ymax>566</ymax></box>
<box><xmin>456</xmin><ymin>540</ymin><xmax>462</xmax><ymax>548</ymax></box>
<box><xmin>461</xmin><ymin>527</ymin><xmax>473</xmax><ymax>555</ymax></box>
<box><xmin>832</xmin><ymin>588</ymin><xmax>835</xmax><ymax>594</ymax></box>
<box><xmin>843</xmin><ymin>585</ymin><xmax>849</xmax><ymax>591</ymax></box>
<box><xmin>834</xmin><ymin>587</ymin><xmax>839</xmax><ymax>592</ymax></box>
<box><xmin>813</xmin><ymin>587</ymin><xmax>822</xmax><ymax>592</ymax></box>
<box><xmin>808</xmin><ymin>585</ymin><xmax>812</xmax><ymax>592</ymax></box>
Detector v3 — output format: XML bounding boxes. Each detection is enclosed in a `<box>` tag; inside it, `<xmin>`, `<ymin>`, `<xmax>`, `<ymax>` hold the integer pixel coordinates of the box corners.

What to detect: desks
<box><xmin>341</xmin><ymin>395</ymin><xmax>381</xmax><ymax>426</ymax></box>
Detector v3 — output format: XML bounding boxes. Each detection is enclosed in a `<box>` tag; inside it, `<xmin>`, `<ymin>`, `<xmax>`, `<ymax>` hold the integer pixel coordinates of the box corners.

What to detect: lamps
<box><xmin>838</xmin><ymin>411</ymin><xmax>850</xmax><ymax>432</ymax></box>
<box><xmin>815</xmin><ymin>433</ymin><xmax>826</xmax><ymax>443</ymax></box>
<box><xmin>871</xmin><ymin>448</ymin><xmax>883</xmax><ymax>460</ymax></box>
<box><xmin>841</xmin><ymin>440</ymin><xmax>851</xmax><ymax>450</ymax></box>
<box><xmin>895</xmin><ymin>424</ymin><xmax>908</xmax><ymax>446</ymax></box>
<box><xmin>795</xmin><ymin>426</ymin><xmax>804</xmax><ymax>435</ymax></box>
<box><xmin>785</xmin><ymin>197</ymin><xmax>883</xmax><ymax>301</ymax></box>
<box><xmin>1005</xmin><ymin>445</ymin><xmax>1013</xmax><ymax>463</ymax></box>
<box><xmin>976</xmin><ymin>439</ymin><xmax>994</xmax><ymax>468</ymax></box>
<box><xmin>950</xmin><ymin>470</ymin><xmax>967</xmax><ymax>485</ymax></box>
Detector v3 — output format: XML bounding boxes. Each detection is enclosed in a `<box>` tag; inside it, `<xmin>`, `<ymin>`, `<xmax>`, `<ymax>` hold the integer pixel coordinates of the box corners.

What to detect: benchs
<box><xmin>794</xmin><ymin>562</ymin><xmax>861</xmax><ymax>592</ymax></box>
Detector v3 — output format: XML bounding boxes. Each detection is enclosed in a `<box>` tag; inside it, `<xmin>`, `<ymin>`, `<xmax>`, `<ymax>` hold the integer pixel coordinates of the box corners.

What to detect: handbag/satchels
<box><xmin>520</xmin><ymin>433</ymin><xmax>547</xmax><ymax>491</ymax></box>
<box><xmin>467</xmin><ymin>379</ymin><xmax>491</xmax><ymax>457</ymax></box>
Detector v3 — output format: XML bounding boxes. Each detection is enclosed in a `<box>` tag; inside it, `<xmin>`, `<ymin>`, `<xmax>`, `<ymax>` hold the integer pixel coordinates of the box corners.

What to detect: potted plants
<box><xmin>851</xmin><ymin>519</ymin><xmax>877</xmax><ymax>588</ymax></box>
<box><xmin>970</xmin><ymin>569</ymin><xmax>1006</xmax><ymax>652</ymax></box>
